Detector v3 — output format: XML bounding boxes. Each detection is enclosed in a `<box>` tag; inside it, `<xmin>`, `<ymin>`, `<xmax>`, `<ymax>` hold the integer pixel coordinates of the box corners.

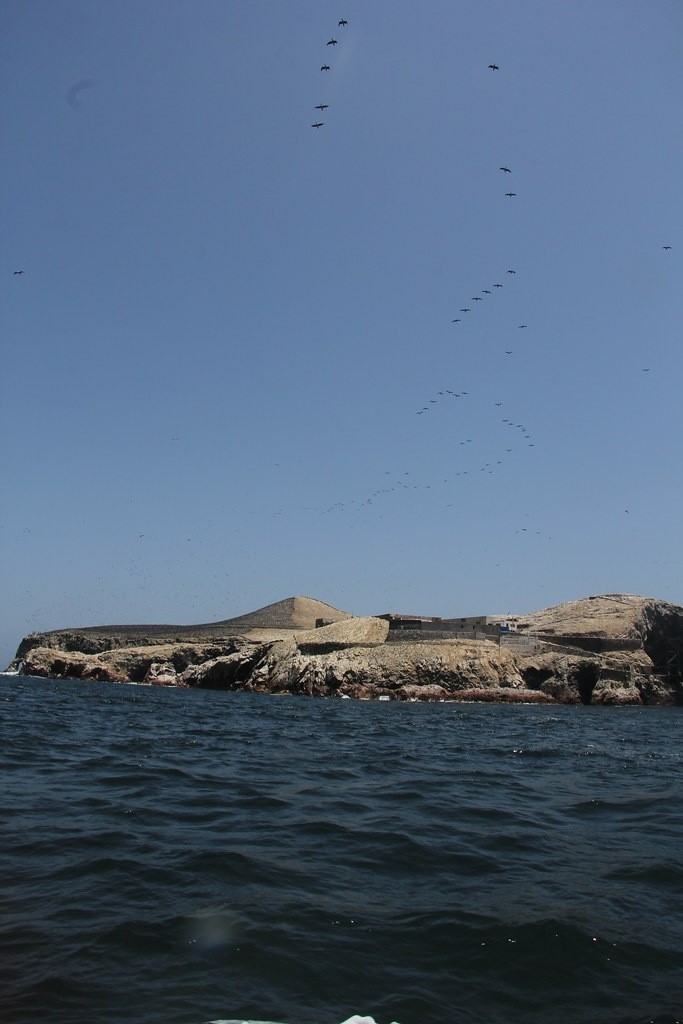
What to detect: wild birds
<box><xmin>488</xmin><ymin>64</ymin><xmax>499</xmax><ymax>71</ymax></box>
<box><xmin>451</xmin><ymin>269</ymin><xmax>515</xmax><ymax>323</ymax></box>
<box><xmin>0</xmin><ymin>391</ymin><xmax>629</xmax><ymax>648</ymax></box>
<box><xmin>519</xmin><ymin>326</ymin><xmax>527</xmax><ymax>329</ymax></box>
<box><xmin>327</xmin><ymin>38</ymin><xmax>338</xmax><ymax>46</ymax></box>
<box><xmin>311</xmin><ymin>122</ymin><xmax>324</xmax><ymax>129</ymax></box>
<box><xmin>14</xmin><ymin>271</ymin><xmax>24</xmax><ymax>275</ymax></box>
<box><xmin>642</xmin><ymin>369</ymin><xmax>650</xmax><ymax>372</ymax></box>
<box><xmin>500</xmin><ymin>166</ymin><xmax>511</xmax><ymax>173</ymax></box>
<box><xmin>321</xmin><ymin>64</ymin><xmax>330</xmax><ymax>72</ymax></box>
<box><xmin>506</xmin><ymin>352</ymin><xmax>512</xmax><ymax>354</ymax></box>
<box><xmin>505</xmin><ymin>192</ymin><xmax>515</xmax><ymax>197</ymax></box>
<box><xmin>662</xmin><ymin>245</ymin><xmax>671</xmax><ymax>250</ymax></box>
<box><xmin>339</xmin><ymin>17</ymin><xmax>348</xmax><ymax>27</ymax></box>
<box><xmin>314</xmin><ymin>104</ymin><xmax>329</xmax><ymax>111</ymax></box>
